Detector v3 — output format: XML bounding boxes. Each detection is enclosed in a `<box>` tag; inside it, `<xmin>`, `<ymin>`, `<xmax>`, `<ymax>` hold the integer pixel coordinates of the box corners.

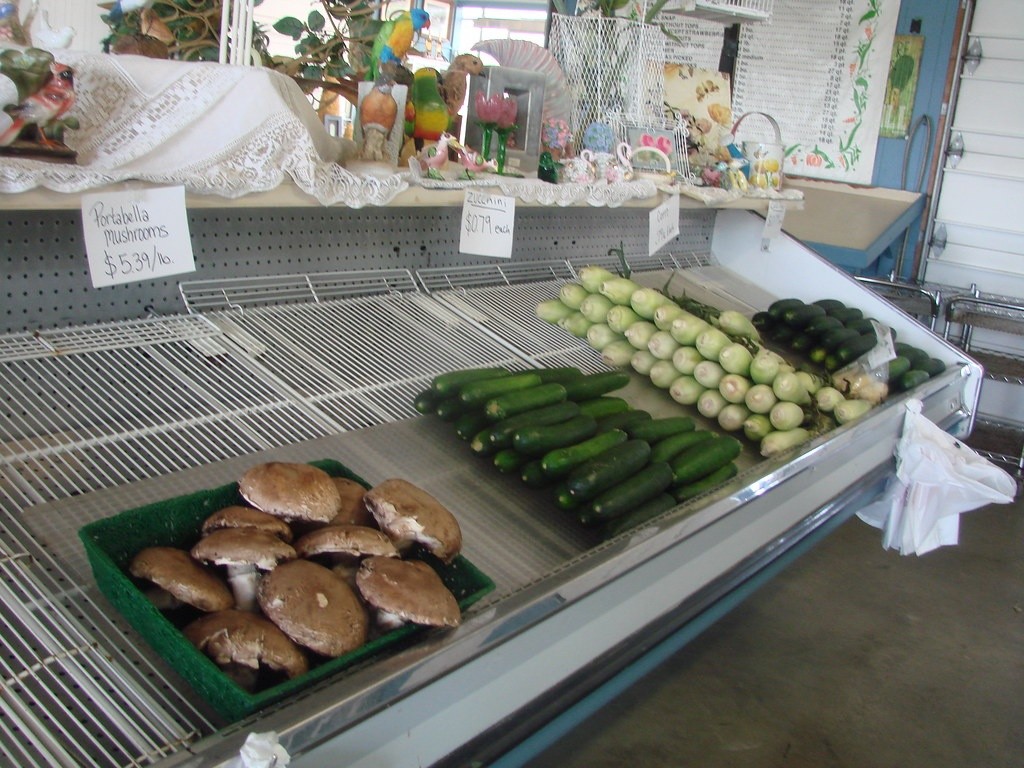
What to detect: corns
<box><xmin>534</xmin><ymin>240</ymin><xmax>890</xmax><ymax>457</ymax></box>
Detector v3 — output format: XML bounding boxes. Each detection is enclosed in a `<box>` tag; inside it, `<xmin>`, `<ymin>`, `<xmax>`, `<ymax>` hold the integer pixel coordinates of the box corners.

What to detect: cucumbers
<box><xmin>752</xmin><ymin>298</ymin><xmax>945</xmax><ymax>391</ymax></box>
<box><xmin>413</xmin><ymin>368</ymin><xmax>742</xmax><ymax>544</ymax></box>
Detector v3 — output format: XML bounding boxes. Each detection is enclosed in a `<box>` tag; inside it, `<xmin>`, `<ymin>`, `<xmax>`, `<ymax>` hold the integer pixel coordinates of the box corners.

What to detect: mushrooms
<box><xmin>131</xmin><ymin>460</ymin><xmax>463</xmax><ymax>691</ymax></box>
